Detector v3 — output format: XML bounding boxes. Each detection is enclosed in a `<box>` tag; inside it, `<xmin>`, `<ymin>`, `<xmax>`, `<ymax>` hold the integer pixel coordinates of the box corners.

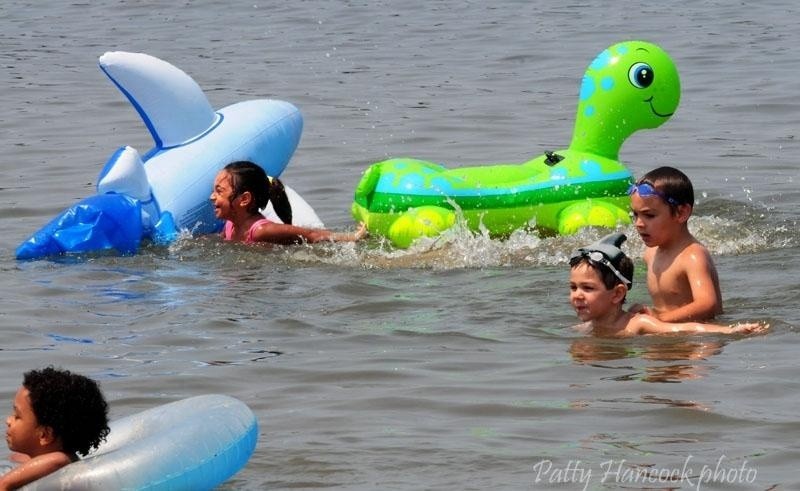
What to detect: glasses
<box><xmin>627</xmin><ymin>184</ymin><xmax>679</xmax><ymax>206</ymax></box>
<box><xmin>570</xmin><ymin>250</ymin><xmax>631</xmax><ymax>286</ymax></box>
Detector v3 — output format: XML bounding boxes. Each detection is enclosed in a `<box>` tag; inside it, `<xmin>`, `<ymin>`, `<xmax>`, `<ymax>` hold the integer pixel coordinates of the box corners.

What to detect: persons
<box><xmin>631</xmin><ymin>164</ymin><xmax>727</xmax><ymax>340</ymax></box>
<box><xmin>567</xmin><ymin>232</ymin><xmax>774</xmax><ymax>340</ymax></box>
<box><xmin>212</xmin><ymin>161</ymin><xmax>371</xmax><ymax>247</ymax></box>
<box><xmin>0</xmin><ymin>364</ymin><xmax>115</xmax><ymax>491</ymax></box>
